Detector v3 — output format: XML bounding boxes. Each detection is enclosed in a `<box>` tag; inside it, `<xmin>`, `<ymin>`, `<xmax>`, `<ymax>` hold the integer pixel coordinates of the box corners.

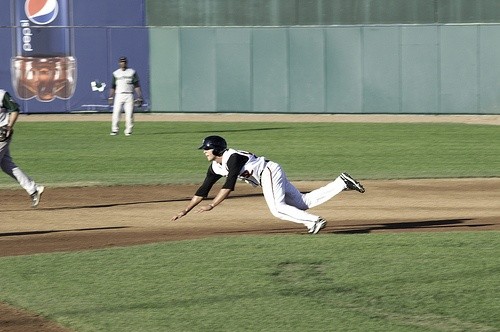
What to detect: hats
<box><xmin>119</xmin><ymin>57</ymin><xmax>127</xmax><ymax>62</ymax></box>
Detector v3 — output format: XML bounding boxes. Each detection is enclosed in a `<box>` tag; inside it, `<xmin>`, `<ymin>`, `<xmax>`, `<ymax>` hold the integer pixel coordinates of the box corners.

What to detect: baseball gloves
<box><xmin>133</xmin><ymin>97</ymin><xmax>144</xmax><ymax>109</ymax></box>
<box><xmin>0</xmin><ymin>126</ymin><xmax>13</xmax><ymax>142</ymax></box>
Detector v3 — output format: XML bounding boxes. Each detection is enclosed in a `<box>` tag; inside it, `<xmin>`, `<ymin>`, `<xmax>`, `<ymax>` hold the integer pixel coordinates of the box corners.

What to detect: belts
<box><xmin>259</xmin><ymin>159</ymin><xmax>270</xmax><ymax>187</ymax></box>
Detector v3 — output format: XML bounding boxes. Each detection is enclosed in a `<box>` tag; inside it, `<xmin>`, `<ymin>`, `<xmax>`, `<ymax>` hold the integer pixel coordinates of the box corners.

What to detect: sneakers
<box><xmin>308</xmin><ymin>217</ymin><xmax>327</xmax><ymax>234</ymax></box>
<box><xmin>27</xmin><ymin>181</ymin><xmax>45</xmax><ymax>207</ymax></box>
<box><xmin>340</xmin><ymin>172</ymin><xmax>366</xmax><ymax>193</ymax></box>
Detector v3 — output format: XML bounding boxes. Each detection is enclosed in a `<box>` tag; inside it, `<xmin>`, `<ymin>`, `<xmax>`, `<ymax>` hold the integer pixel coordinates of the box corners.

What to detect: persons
<box><xmin>108</xmin><ymin>57</ymin><xmax>143</xmax><ymax>136</ymax></box>
<box><xmin>0</xmin><ymin>89</ymin><xmax>44</xmax><ymax>208</ymax></box>
<box><xmin>170</xmin><ymin>135</ymin><xmax>365</xmax><ymax>235</ymax></box>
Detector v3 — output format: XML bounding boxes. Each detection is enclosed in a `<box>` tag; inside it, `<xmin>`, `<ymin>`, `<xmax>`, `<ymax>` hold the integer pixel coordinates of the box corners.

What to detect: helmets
<box><xmin>198</xmin><ymin>135</ymin><xmax>227</xmax><ymax>157</ymax></box>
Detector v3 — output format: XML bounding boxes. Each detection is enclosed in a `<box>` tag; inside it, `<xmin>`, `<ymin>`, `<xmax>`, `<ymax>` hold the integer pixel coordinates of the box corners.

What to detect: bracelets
<box><xmin>108</xmin><ymin>97</ymin><xmax>113</xmax><ymax>99</ymax></box>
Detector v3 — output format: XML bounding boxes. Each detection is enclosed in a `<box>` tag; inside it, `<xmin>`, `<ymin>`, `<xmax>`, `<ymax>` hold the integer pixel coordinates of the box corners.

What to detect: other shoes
<box><xmin>125</xmin><ymin>133</ymin><xmax>132</xmax><ymax>136</ymax></box>
<box><xmin>110</xmin><ymin>132</ymin><xmax>119</xmax><ymax>136</ymax></box>
